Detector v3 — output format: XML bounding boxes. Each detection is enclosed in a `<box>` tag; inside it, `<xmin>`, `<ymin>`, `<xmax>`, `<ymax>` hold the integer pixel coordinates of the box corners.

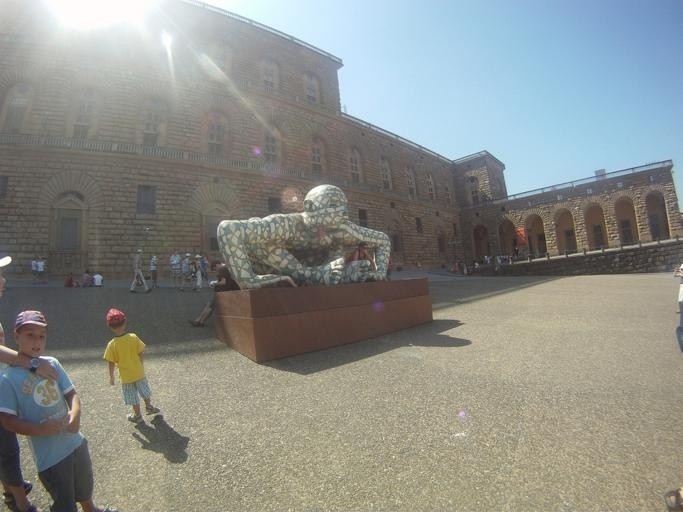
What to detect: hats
<box><xmin>106</xmin><ymin>308</ymin><xmax>126</xmax><ymax>324</ymax></box>
<box><xmin>185</xmin><ymin>253</ymin><xmax>192</xmax><ymax>257</ymax></box>
<box><xmin>12</xmin><ymin>309</ymin><xmax>47</xmax><ymax>333</ymax></box>
<box><xmin>194</xmin><ymin>255</ymin><xmax>202</xmax><ymax>258</ymax></box>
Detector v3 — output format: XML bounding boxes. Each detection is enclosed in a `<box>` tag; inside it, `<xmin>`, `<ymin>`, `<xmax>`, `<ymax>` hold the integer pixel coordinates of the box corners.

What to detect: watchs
<box><xmin>29</xmin><ymin>357</ymin><xmax>40</xmax><ymax>372</ymax></box>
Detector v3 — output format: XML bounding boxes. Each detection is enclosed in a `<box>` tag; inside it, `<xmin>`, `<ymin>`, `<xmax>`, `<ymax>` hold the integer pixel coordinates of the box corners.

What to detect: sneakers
<box><xmin>126</xmin><ymin>413</ymin><xmax>143</xmax><ymax>422</ymax></box>
<box><xmin>186</xmin><ymin>317</ymin><xmax>207</xmax><ymax>328</ymax></box>
<box><xmin>3</xmin><ymin>480</ymin><xmax>32</xmax><ymax>504</ymax></box>
<box><xmin>145</xmin><ymin>406</ymin><xmax>160</xmax><ymax>416</ymax></box>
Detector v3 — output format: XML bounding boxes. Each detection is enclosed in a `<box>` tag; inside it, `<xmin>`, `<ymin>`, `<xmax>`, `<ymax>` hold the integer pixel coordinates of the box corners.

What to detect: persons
<box><xmin>36</xmin><ymin>256</ymin><xmax>44</xmax><ymax>285</ymax></box>
<box><xmin>62</xmin><ymin>272</ymin><xmax>73</xmax><ymax>288</ymax></box>
<box><xmin>41</xmin><ymin>255</ymin><xmax>49</xmax><ymax>284</ymax></box>
<box><xmin>0</xmin><ymin>309</ymin><xmax>118</xmax><ymax>512</ymax></box>
<box><xmin>101</xmin><ymin>306</ymin><xmax>159</xmax><ymax>422</ymax></box>
<box><xmin>664</xmin><ymin>486</ymin><xmax>682</xmax><ymax>512</ymax></box>
<box><xmin>0</xmin><ymin>254</ymin><xmax>58</xmax><ymax>512</ymax></box>
<box><xmin>91</xmin><ymin>271</ymin><xmax>104</xmax><ymax>287</ymax></box>
<box><xmin>672</xmin><ymin>263</ymin><xmax>683</xmax><ymax>328</ymax></box>
<box><xmin>80</xmin><ymin>270</ymin><xmax>92</xmax><ymax>288</ymax></box>
<box><xmin>188</xmin><ymin>258</ymin><xmax>231</xmax><ymax>328</ymax></box>
<box><xmin>215</xmin><ymin>185</ymin><xmax>392</xmax><ymax>290</ymax></box>
<box><xmin>149</xmin><ymin>249</ymin><xmax>208</xmax><ymax>295</ymax></box>
<box><xmin>129</xmin><ymin>248</ymin><xmax>152</xmax><ymax>294</ymax></box>
<box><xmin>30</xmin><ymin>257</ymin><xmax>37</xmax><ymax>284</ymax></box>
